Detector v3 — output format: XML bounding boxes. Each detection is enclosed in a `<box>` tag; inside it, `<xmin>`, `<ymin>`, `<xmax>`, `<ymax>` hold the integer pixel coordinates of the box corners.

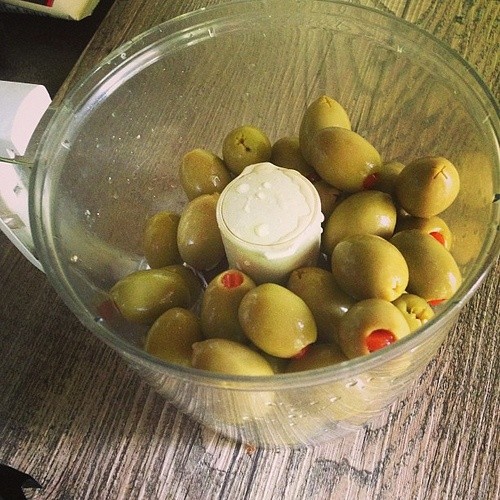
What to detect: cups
<box><xmin>0</xmin><ymin>0</ymin><xmax>500</xmax><ymax>449</ymax></box>
